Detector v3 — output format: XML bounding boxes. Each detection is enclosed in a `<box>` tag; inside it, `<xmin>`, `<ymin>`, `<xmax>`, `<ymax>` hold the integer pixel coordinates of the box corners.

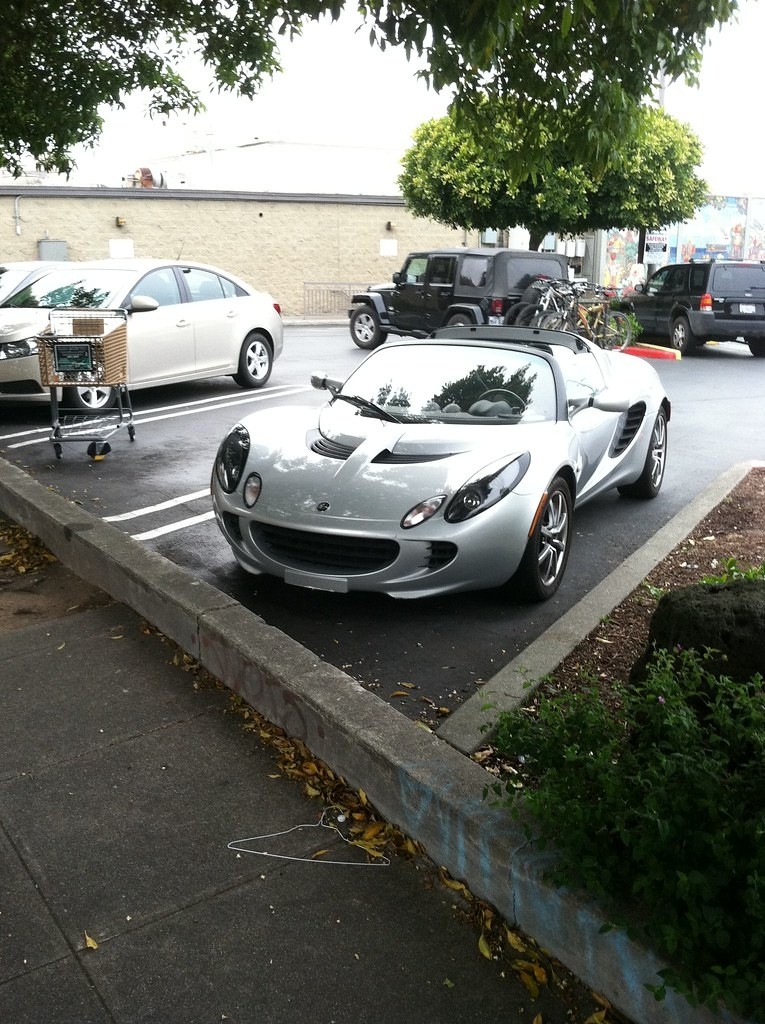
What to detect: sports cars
<box><xmin>210</xmin><ymin>322</ymin><xmax>672</xmax><ymax>601</ymax></box>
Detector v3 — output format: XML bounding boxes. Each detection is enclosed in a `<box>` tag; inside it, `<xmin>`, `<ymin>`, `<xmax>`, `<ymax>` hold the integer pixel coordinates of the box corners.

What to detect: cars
<box><xmin>1</xmin><ymin>257</ymin><xmax>284</xmax><ymax>415</ymax></box>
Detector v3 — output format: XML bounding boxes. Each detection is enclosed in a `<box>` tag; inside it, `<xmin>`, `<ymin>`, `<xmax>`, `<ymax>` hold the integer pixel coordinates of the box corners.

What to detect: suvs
<box><xmin>348</xmin><ymin>247</ymin><xmax>569</xmax><ymax>349</ymax></box>
<box><xmin>621</xmin><ymin>257</ymin><xmax>765</xmax><ymax>358</ymax></box>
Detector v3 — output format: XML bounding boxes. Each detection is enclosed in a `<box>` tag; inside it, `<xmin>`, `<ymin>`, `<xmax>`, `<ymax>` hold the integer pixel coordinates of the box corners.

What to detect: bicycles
<box><xmin>502</xmin><ymin>275</ymin><xmax>632</xmax><ymax>353</ymax></box>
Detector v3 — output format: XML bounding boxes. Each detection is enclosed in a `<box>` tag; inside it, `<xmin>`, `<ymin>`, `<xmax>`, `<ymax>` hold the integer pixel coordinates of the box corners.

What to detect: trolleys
<box><xmin>36</xmin><ymin>308</ymin><xmax>136</xmax><ymax>462</ymax></box>
